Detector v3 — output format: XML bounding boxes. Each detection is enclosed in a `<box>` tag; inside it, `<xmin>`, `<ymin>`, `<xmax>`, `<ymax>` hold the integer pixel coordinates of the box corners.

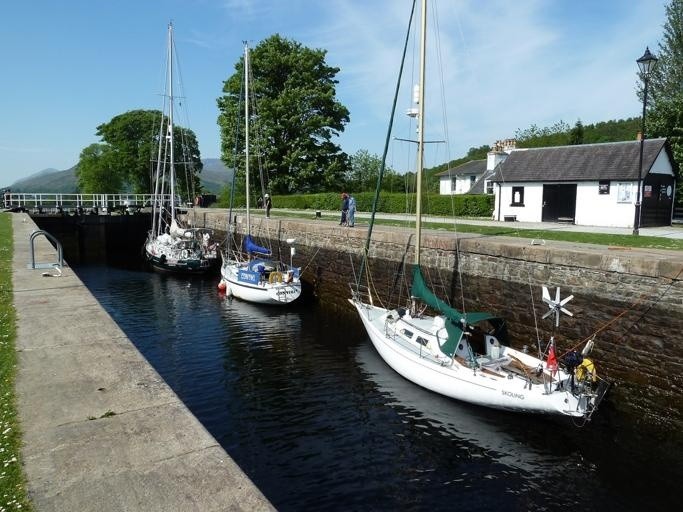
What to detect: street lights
<box><xmin>633</xmin><ymin>47</ymin><xmax>659</xmax><ymax>239</ymax></box>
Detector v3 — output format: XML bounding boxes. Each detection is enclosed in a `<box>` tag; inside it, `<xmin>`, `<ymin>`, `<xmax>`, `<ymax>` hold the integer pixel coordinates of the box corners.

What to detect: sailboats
<box><xmin>142</xmin><ymin>18</ymin><xmax>219</xmax><ymax>274</ymax></box>
<box><xmin>348</xmin><ymin>1</ymin><xmax>608</xmax><ymax>417</ymax></box>
<box><xmin>219</xmin><ymin>41</ymin><xmax>301</xmax><ymax>305</ymax></box>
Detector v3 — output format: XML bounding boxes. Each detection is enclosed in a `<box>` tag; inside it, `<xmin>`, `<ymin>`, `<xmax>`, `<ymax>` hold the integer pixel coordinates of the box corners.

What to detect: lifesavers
<box><xmin>269</xmin><ymin>272</ymin><xmax>282</xmax><ymax>284</ymax></box>
<box><xmin>181</xmin><ymin>250</ymin><xmax>188</xmax><ymax>258</ymax></box>
<box><xmin>576</xmin><ymin>359</ymin><xmax>596</xmax><ymax>384</ymax></box>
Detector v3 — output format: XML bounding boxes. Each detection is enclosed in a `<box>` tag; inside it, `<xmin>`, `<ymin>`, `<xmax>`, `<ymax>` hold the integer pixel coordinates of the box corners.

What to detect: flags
<box><xmin>547</xmin><ymin>345</ymin><xmax>558</xmax><ymax>376</ymax></box>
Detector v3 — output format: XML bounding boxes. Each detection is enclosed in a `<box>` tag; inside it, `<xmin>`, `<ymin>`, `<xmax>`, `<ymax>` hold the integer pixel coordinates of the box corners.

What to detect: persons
<box><xmin>2</xmin><ymin>189</ymin><xmax>11</xmax><ymax>208</ymax></box>
<box><xmin>338</xmin><ymin>193</ymin><xmax>349</xmax><ymax>225</ymax></box>
<box><xmin>262</xmin><ymin>194</ymin><xmax>272</xmax><ymax>219</ymax></box>
<box><xmin>346</xmin><ymin>195</ymin><xmax>357</xmax><ymax>227</ymax></box>
